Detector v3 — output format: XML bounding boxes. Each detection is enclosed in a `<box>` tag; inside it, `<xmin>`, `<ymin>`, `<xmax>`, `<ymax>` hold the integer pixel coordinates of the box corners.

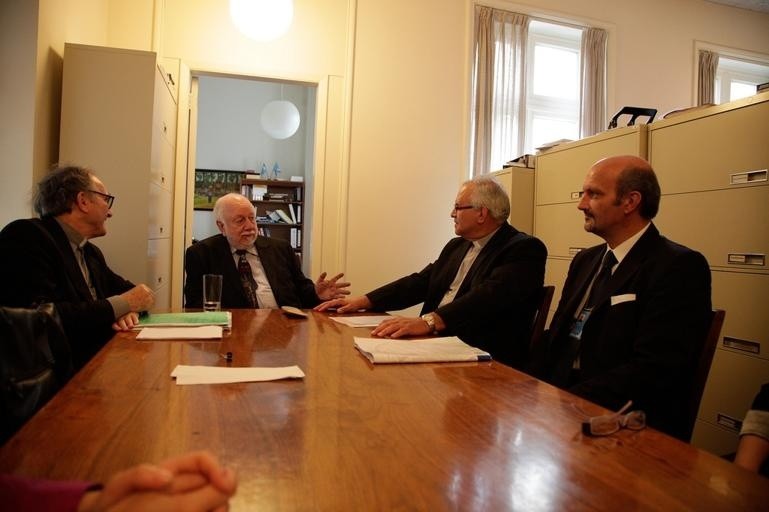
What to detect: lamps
<box><xmin>261</xmin><ymin>84</ymin><xmax>301</xmax><ymax>141</ymax></box>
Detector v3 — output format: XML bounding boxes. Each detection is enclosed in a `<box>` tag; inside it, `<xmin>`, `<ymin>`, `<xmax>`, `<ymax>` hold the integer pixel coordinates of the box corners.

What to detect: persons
<box><xmin>525</xmin><ymin>154</ymin><xmax>713</xmax><ymax>443</ymax></box>
<box><xmin>0</xmin><ymin>448</ymin><xmax>243</xmax><ymax>512</ymax></box>
<box><xmin>183</xmin><ymin>192</ymin><xmax>352</xmax><ymax>310</ymax></box>
<box><xmin>733</xmin><ymin>380</ymin><xmax>769</xmax><ymax>474</ymax></box>
<box><xmin>0</xmin><ymin>163</ymin><xmax>156</xmax><ymax>387</ymax></box>
<box><xmin>312</xmin><ymin>175</ymin><xmax>549</xmax><ymax>370</ymax></box>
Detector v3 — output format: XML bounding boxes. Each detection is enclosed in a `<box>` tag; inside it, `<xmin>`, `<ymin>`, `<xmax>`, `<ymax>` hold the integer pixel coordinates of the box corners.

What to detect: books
<box><xmin>535</xmin><ymin>139</ymin><xmax>573</xmax><ymax>151</ymax></box>
<box><xmin>241</xmin><ymin>173</ymin><xmax>304</xmax><ymax>257</ymax></box>
<box><xmin>131</xmin><ymin>310</ymin><xmax>233</xmax><ymax>332</ymax></box>
<box><xmin>351</xmin><ymin>333</ymin><xmax>493</xmax><ymax>365</ymax></box>
<box><xmin>501</xmin><ymin>154</ymin><xmax>534</xmax><ymax>170</ymax></box>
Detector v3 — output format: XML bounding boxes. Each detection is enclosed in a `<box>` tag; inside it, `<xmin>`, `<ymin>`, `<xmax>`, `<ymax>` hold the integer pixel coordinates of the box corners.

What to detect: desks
<box><xmin>0</xmin><ymin>309</ymin><xmax>767</xmax><ymax>512</ymax></box>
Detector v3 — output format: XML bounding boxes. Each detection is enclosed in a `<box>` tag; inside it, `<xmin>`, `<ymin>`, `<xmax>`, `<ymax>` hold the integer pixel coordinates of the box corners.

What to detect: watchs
<box><xmin>420</xmin><ymin>313</ymin><xmax>436</xmax><ymax>332</ymax></box>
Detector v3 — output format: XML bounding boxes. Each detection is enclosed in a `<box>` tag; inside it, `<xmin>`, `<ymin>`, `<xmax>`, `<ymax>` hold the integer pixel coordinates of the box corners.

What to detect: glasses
<box><xmin>87</xmin><ymin>189</ymin><xmax>114</xmax><ymax>209</ymax></box>
<box><xmin>455</xmin><ymin>204</ymin><xmax>473</xmax><ymax>211</ymax></box>
<box><xmin>572</xmin><ymin>401</ymin><xmax>646</xmax><ymax>437</ymax></box>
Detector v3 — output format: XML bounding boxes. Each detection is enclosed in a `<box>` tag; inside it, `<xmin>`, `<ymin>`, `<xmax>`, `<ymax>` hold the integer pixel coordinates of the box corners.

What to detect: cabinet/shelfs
<box><xmin>239</xmin><ymin>180</ymin><xmax>305</xmax><ymax>269</ymax></box>
<box><xmin>60</xmin><ymin>42</ymin><xmax>177</xmax><ymax>318</ymax></box>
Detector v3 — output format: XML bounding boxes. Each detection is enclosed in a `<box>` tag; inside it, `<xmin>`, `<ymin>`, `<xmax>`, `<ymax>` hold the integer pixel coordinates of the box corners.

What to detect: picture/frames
<box><xmin>195</xmin><ymin>166</ymin><xmax>252</xmax><ymax>213</ymax></box>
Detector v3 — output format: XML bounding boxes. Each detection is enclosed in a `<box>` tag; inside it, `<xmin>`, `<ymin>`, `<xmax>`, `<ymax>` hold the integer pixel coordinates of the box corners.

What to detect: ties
<box><xmin>585</xmin><ymin>250</ymin><xmax>618</xmax><ymax>310</ymax></box>
<box><xmin>236</xmin><ymin>249</ymin><xmax>258</xmax><ymax>308</ymax></box>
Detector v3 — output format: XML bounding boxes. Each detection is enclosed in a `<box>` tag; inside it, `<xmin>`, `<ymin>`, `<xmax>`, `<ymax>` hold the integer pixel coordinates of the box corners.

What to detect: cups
<box><xmin>203</xmin><ymin>274</ymin><xmax>223</xmax><ymax>312</ymax></box>
